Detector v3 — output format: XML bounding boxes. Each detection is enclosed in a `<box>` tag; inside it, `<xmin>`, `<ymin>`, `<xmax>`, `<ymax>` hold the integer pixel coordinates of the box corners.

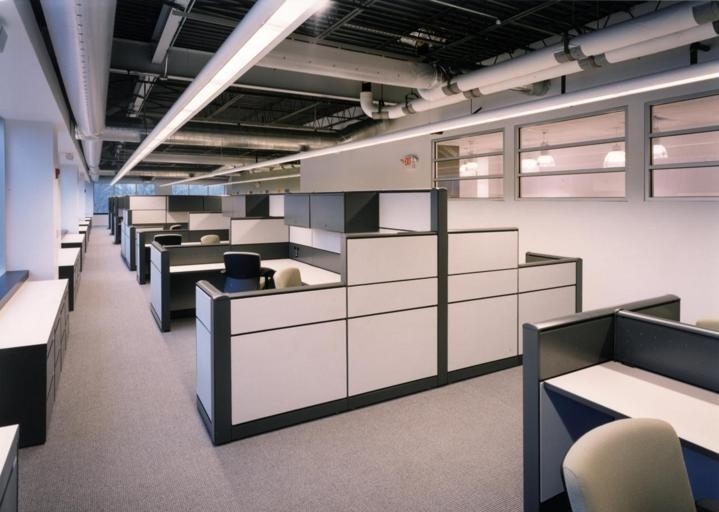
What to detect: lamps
<box><xmin>536</xmin><ymin>131</ymin><xmax>556</xmax><ymax>169</ymax></box>
<box><xmin>604</xmin><ymin>117</ymin><xmax>665</xmax><ymax>173</ymax></box>
<box><xmin>107</xmin><ymin>0</ymin><xmax>326</xmax><ymax>187</ymax></box>
<box><xmin>462</xmin><ymin>143</ymin><xmax>478</xmax><ymax>171</ymax></box>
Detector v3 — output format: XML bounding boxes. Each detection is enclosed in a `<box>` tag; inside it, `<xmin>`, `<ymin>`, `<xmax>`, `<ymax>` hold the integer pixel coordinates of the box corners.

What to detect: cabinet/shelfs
<box><xmin>0</xmin><ymin>214</ymin><xmax>93</xmax><ymax>512</ymax></box>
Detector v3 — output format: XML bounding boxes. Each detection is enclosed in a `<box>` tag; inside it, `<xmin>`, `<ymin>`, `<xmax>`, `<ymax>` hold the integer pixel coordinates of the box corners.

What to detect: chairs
<box><xmin>560</xmin><ymin>417</ymin><xmax>698</xmax><ymax>512</ymax></box>
<box><xmin>694</xmin><ymin>317</ymin><xmax>719</xmax><ymax>333</ymax></box>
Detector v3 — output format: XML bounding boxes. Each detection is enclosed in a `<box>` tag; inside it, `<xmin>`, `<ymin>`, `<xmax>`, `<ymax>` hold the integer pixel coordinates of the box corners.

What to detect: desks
<box><xmin>107</xmin><ymin>187</ymin><xmax>583</xmax><ymax>447</ymax></box>
<box><xmin>523</xmin><ymin>294</ymin><xmax>718</xmax><ymax>511</ymax></box>
